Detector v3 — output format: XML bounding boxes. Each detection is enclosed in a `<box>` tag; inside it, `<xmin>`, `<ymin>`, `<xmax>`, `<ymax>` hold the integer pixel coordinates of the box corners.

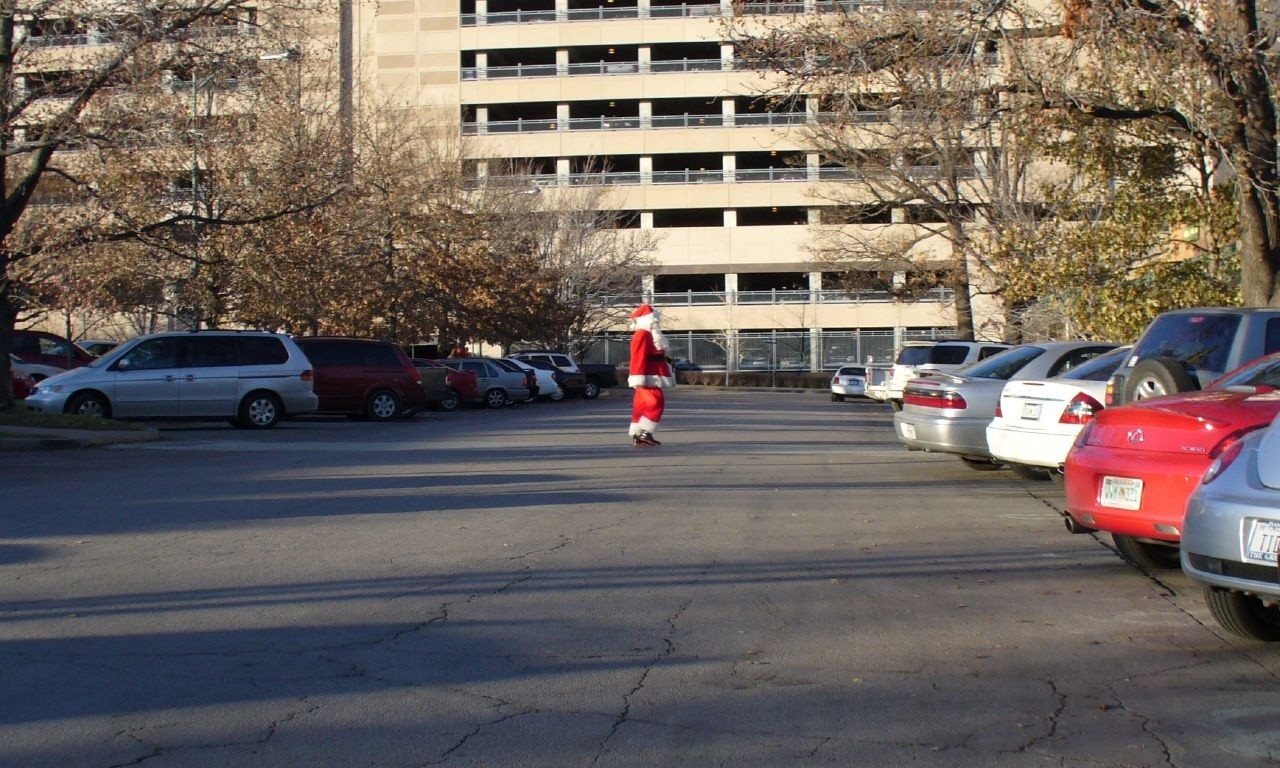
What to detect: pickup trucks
<box><xmin>865</xmin><ymin>355</ymin><xmax>895</xmax><ymax>404</ymax></box>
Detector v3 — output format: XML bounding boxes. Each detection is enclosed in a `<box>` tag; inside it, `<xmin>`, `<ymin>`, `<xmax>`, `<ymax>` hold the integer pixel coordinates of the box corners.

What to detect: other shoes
<box><xmin>633</xmin><ymin>434</ymin><xmax>645</xmax><ymax>448</ymax></box>
<box><xmin>638</xmin><ymin>430</ymin><xmax>661</xmax><ymax>446</ymax></box>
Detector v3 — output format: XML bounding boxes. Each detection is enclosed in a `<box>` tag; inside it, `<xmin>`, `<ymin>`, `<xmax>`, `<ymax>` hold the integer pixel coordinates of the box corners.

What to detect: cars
<box><xmin>1179</xmin><ymin>409</ymin><xmax>1280</xmax><ymax>640</ymax></box>
<box><xmin>671</xmin><ymin>358</ymin><xmax>702</xmax><ymax>372</ymax></box>
<box><xmin>401</xmin><ymin>342</ymin><xmax>618</xmax><ymax>413</ymax></box>
<box><xmin>985</xmin><ymin>343</ymin><xmax>1135</xmax><ymax>482</ymax></box>
<box><xmin>893</xmin><ymin>340</ymin><xmax>1123</xmax><ymax>471</ymax></box>
<box><xmin>778</xmin><ymin>351</ymin><xmax>807</xmax><ymax>370</ymax></box>
<box><xmin>1064</xmin><ymin>350</ymin><xmax>1280</xmax><ymax>570</ymax></box>
<box><xmin>829</xmin><ymin>366</ymin><xmax>866</xmax><ymax>403</ymax></box>
<box><xmin>10</xmin><ymin>329</ymin><xmax>153</xmax><ymax>399</ymax></box>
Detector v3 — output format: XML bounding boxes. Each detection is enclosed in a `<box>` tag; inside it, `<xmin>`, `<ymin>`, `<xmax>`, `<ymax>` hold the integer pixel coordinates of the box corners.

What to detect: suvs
<box><xmin>823</xmin><ymin>343</ymin><xmax>856</xmax><ymax>368</ymax></box>
<box><xmin>290</xmin><ymin>335</ymin><xmax>426</xmax><ymax>422</ymax></box>
<box><xmin>738</xmin><ymin>348</ymin><xmax>770</xmax><ymax>369</ymax></box>
<box><xmin>887</xmin><ymin>339</ymin><xmax>1018</xmax><ymax>412</ymax></box>
<box><xmin>23</xmin><ymin>329</ymin><xmax>321</xmax><ymax>431</ymax></box>
<box><xmin>1104</xmin><ymin>306</ymin><xmax>1280</xmax><ymax>409</ymax></box>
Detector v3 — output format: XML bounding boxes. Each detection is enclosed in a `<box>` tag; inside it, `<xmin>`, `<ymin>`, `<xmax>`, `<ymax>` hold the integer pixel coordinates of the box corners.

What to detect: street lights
<box><xmin>189</xmin><ymin>53</ymin><xmax>291</xmax><ymax>331</ymax></box>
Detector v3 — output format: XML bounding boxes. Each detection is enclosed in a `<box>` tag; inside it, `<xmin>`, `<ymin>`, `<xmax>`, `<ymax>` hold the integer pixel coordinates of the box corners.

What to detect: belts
<box><xmin>648</xmin><ymin>353</ymin><xmax>665</xmax><ymax>363</ymax></box>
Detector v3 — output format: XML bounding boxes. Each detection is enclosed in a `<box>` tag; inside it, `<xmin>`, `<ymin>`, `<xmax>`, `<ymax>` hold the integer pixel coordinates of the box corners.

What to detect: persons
<box><xmin>630</xmin><ymin>305</ymin><xmax>674</xmax><ymax>447</ymax></box>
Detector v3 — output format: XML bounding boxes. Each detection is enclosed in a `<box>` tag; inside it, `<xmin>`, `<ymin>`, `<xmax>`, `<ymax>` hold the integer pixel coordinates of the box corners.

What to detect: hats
<box><xmin>631</xmin><ymin>305</ymin><xmax>658</xmax><ymax>325</ymax></box>
